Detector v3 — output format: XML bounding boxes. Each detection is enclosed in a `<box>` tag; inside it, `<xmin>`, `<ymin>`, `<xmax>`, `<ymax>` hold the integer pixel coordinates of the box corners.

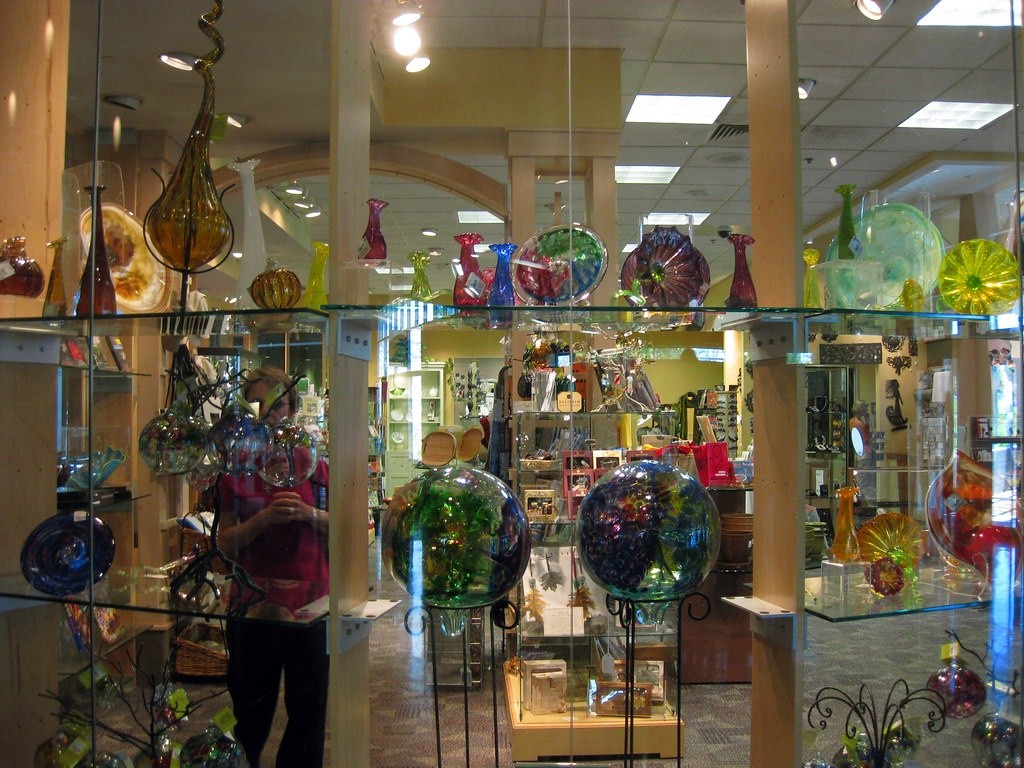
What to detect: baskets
<box><xmin>181</xmin><ymin>529</ymin><xmax>233</xmax><ymax>575</ymax></box>
<box><xmin>174</xmin><ymin>621</ymin><xmax>230</xmax><ymax>676</ymax></box>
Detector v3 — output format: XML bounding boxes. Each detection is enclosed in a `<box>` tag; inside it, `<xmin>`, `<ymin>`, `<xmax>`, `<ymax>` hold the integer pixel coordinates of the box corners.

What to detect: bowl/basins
<box><xmin>804</xmin><ymin>521</ymin><xmax>828</xmax><ymax>562</ymax></box>
<box><xmin>715</xmin><ymin>512</ymin><xmax>754</xmax><ymax>570</ymax></box>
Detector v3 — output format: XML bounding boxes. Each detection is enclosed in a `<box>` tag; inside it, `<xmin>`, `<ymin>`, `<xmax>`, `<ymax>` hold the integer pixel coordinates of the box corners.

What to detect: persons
<box><xmin>849</xmin><ymin>400</ymin><xmax>877</xmax><ymax>507</ymax></box>
<box><xmin>216</xmin><ymin>367</ymin><xmax>329</xmax><ymax>768</ymax></box>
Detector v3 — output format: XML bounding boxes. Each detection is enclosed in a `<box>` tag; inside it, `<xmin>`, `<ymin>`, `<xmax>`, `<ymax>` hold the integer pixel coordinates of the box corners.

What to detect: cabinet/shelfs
<box><xmin>514</xmin><ymin>409</ymin><xmax>680</xmax><ymax>722</ymax></box>
<box><xmin>385</xmin><ymin>361</ymin><xmax>444</xmax><ymax>498</ymax></box>
<box><xmin>743</xmin><ymin>308</ymin><xmax>1022</xmax><ymax>624</ymax></box>
<box><xmin>704</xmin><ymin>486</ymin><xmax>832</xmax><ymax>574</ymax></box>
<box><xmin>425</xmin><ymin>608</ymin><xmax>484</xmax><ymax>689</ymax></box>
<box><xmin>1</xmin><ymin>307</ymin><xmax>331</xmax><ymax>656</ymax></box>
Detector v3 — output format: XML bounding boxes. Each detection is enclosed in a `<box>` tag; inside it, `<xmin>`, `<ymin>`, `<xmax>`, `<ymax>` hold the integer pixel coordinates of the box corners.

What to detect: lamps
<box><xmin>391</xmin><ymin>0</ymin><xmax>429</xmax><ymax>73</ymax></box>
<box><xmin>421</xmin><ymin>228</ymin><xmax>437</xmax><ymax>237</ymax></box>
<box><xmin>853</xmin><ymin>0</ymin><xmax>896</xmax><ymax>21</ymax></box>
<box><xmin>227</xmin><ymin>113</ymin><xmax>248</xmax><ymax>128</ymax></box>
<box><xmin>159</xmin><ymin>52</ymin><xmax>197</xmax><ymax>71</ymax></box>
<box><xmin>798</xmin><ymin>79</ymin><xmax>815</xmax><ymax>100</ymax></box>
<box><xmin>285</xmin><ymin>179</ymin><xmax>322</xmax><ymax>218</ymax></box>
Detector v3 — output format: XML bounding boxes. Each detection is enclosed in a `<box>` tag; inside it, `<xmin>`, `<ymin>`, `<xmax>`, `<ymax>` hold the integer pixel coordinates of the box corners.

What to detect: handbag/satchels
<box><xmin>692</xmin><ymin>442</ymin><xmax>731</xmax><ymax>487</ymax></box>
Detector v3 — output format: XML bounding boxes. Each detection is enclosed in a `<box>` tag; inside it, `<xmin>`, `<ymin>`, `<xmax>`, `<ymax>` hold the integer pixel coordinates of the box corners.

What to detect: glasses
<box><xmin>252</xmin><ymin>400</ymin><xmax>292</xmax><ymax>410</ymax></box>
<box><xmin>717</xmin><ymin>394</ymin><xmax>739</xmax><ymax>442</ymax></box>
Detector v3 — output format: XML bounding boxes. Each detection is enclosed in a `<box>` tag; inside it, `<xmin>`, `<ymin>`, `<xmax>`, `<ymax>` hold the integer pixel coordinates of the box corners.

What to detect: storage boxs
<box><xmin>596</xmin><ymin>660</ymin><xmax>664</xmax><ymax>717</ymax></box>
<box><xmin>523</xmin><ymin>659</ymin><xmax>566</xmax><ymax>716</ymax></box>
<box><xmin>520</xmin><ymin>435</ymin><xmax>671</xmax><ymax>522</ymax></box>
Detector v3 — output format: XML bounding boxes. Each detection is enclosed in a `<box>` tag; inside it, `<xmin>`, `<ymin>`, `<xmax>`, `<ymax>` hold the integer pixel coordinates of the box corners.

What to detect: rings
<box><xmin>289</xmin><ymin>508</ymin><xmax>295</xmax><ymax>513</ymax></box>
